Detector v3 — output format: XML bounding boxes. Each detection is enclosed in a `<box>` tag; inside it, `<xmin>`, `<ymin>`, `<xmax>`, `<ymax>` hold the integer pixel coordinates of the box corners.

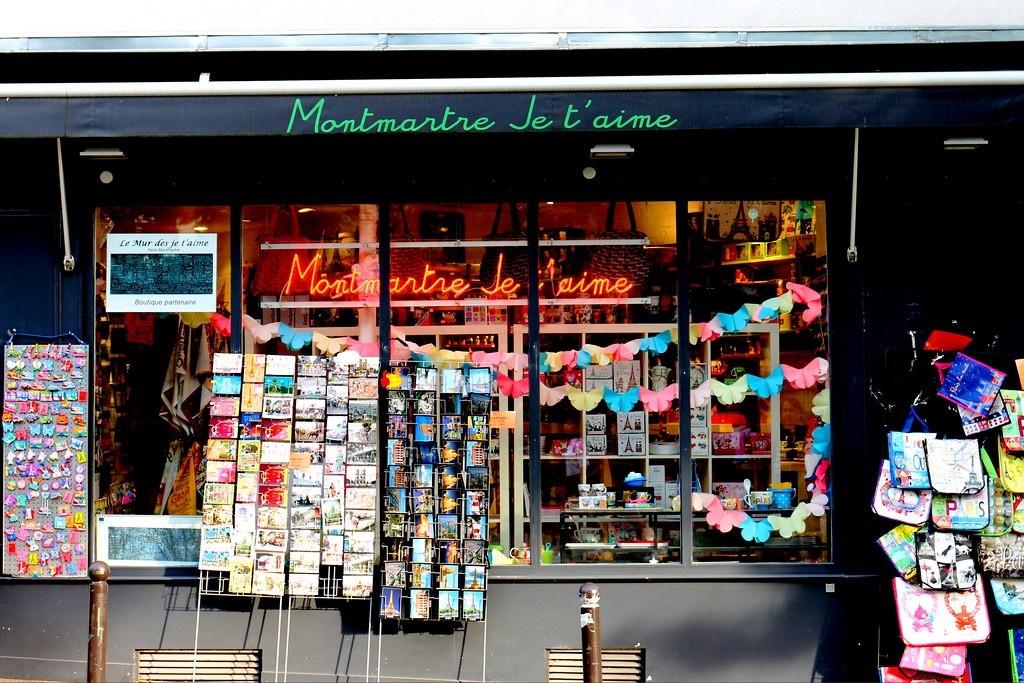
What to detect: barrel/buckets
<box><xmin>769</xmin><ymin>487</ymin><xmax>795</xmax><ymax>507</ymax></box>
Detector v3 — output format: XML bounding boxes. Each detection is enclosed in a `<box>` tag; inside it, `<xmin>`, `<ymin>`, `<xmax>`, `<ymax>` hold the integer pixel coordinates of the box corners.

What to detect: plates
<box><xmin>618</xmin><ymin>543</ymin><xmax>655</xmax><ymax>549</ymax></box>
<box><xmin>569</xmin><ymin>542</ymin><xmax>616</xmax><ymax>549</ymax></box>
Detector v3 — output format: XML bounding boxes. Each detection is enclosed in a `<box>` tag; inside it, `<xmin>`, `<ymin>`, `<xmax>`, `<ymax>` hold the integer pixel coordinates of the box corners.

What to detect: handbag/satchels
<box><xmin>257</xmin><ymin>207</ymin><xmax>322</xmax><ymax>295</ymax></box>
<box><xmin>877</xmin><ymin>351</ymin><xmax>1024</xmax><ymax>683</ymax></box>
<box><xmin>383</xmin><ymin>204</ymin><xmax>446</xmax><ymax>298</ymax></box>
<box><xmin>589</xmin><ymin>200</ymin><xmax>650</xmax><ymax>293</ymax></box>
<box><xmin>480</xmin><ymin>201</ymin><xmax>532</xmax><ymax>289</ymax></box>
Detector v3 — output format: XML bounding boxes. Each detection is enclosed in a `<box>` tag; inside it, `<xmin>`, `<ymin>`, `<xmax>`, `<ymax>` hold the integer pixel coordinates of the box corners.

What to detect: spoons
<box><xmin>573</xmin><ymin>516</ymin><xmax>580</xmax><ymax>538</ymax></box>
<box><xmin>744</xmin><ymin>479</ymin><xmax>751</xmax><ymax>508</ymax></box>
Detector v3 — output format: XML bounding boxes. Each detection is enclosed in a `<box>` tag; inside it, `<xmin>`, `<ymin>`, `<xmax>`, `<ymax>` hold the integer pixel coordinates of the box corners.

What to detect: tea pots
<box><xmin>648</xmin><ymin>362</ymin><xmax>671</xmax><ymax>392</ymax></box>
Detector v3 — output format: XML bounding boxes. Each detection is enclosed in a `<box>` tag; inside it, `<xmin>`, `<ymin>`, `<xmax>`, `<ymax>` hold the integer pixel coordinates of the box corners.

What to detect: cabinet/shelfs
<box><xmin>512</xmin><ymin>322</ymin><xmax>779</xmax><ymax>552</ymax></box>
<box><xmin>244</xmin><ymin>318</ymin><xmax>510</xmax><ymax>559</ymax></box>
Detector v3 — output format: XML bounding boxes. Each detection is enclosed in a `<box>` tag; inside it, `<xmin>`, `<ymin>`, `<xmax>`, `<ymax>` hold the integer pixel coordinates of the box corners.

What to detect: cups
<box><xmin>592</xmin><ymin>484</ymin><xmax>606</xmax><ymax>496</ymax></box>
<box><xmin>607</xmin><ymin>492</ymin><xmax>616</xmax><ymax>508</ymax></box>
<box><xmin>565</xmin><ymin>499</ymin><xmax>579</xmax><ymax>510</ymax></box>
<box><xmin>593</xmin><ymin>499</ymin><xmax>600</xmax><ymax>507</ymax></box>
<box><xmin>731</xmin><ymin>367</ymin><xmax>745</xmax><ymax>379</ymax></box>
<box><xmin>582</xmin><ymin>499</ymin><xmax>590</xmax><ymax>507</ymax></box>
<box><xmin>510</xmin><ymin>543</ymin><xmax>530</xmax><ymax>564</ymax></box>
<box><xmin>642</xmin><ymin>528</ymin><xmax>655</xmax><ymax>542</ymax></box>
<box><xmin>751</xmin><ymin>432</ymin><xmax>772</xmax><ymax>453</ymax></box>
<box><xmin>574</xmin><ymin>528</ymin><xmax>601</xmax><ymax>542</ymax></box>
<box><xmin>743</xmin><ymin>492</ymin><xmax>773</xmax><ymax>509</ymax></box>
<box><xmin>579</xmin><ymin>484</ymin><xmax>591</xmax><ymax>496</ymax></box>
<box><xmin>637</xmin><ymin>492</ymin><xmax>651</xmax><ymax>502</ymax></box>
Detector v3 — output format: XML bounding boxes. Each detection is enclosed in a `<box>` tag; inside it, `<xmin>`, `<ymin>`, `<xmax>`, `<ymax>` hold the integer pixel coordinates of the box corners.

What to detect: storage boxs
<box><xmin>583</xmin><ymin>359</ymin><xmax>775</xmax><ymax>509</ymax></box>
<box><xmin>721</xmin><ymin>231</ymin><xmax>817</xmax><ymax>261</ymax></box>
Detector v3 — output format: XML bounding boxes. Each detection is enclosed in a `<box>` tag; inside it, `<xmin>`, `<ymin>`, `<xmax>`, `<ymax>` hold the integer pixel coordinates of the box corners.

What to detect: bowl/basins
<box><xmin>720</xmin><ymin>501</ymin><xmax>736</xmax><ymax>510</ymax></box>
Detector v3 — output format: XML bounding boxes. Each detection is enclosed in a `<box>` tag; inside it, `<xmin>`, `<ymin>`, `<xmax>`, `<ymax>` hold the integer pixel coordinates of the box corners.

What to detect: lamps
<box><xmin>79</xmin><ymin>147</ymin><xmax>128</xmax><ymax>160</ymax></box>
<box><xmin>943</xmin><ymin>136</ymin><xmax>989</xmax><ymax>152</ymax></box>
<box><xmin>589</xmin><ymin>142</ymin><xmax>635</xmax><ymax>160</ymax></box>
